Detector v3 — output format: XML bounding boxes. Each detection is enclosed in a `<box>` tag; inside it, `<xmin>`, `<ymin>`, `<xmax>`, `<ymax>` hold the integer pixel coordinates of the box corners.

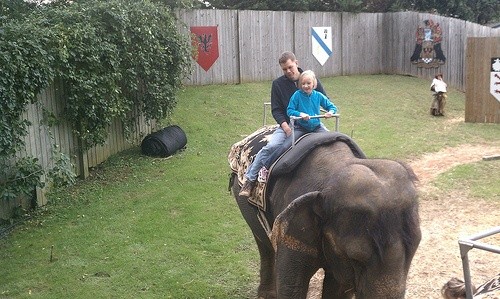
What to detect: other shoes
<box><xmin>237</xmin><ymin>181</ymin><xmax>252</xmax><ymax>197</ymax></box>
<box><xmin>440</xmin><ymin>110</ymin><xmax>444</xmax><ymax>116</ymax></box>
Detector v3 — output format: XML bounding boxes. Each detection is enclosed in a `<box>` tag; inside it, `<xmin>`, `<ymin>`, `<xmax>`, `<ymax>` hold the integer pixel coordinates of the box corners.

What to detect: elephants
<box><xmin>227</xmin><ymin>124</ymin><xmax>424</xmax><ymax>298</ymax></box>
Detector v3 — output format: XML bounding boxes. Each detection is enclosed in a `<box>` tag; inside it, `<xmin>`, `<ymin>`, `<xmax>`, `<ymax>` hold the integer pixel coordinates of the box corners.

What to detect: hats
<box><xmin>435</xmin><ymin>73</ymin><xmax>442</xmax><ymax>78</ymax></box>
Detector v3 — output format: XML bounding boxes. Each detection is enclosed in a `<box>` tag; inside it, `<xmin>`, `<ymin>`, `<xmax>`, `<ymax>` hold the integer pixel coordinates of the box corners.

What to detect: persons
<box><xmin>258</xmin><ymin>70</ymin><xmax>337</xmax><ymax>183</ymax></box>
<box><xmin>431</xmin><ymin>73</ymin><xmax>448</xmax><ymax>116</ymax></box>
<box><xmin>239</xmin><ymin>51</ymin><xmax>331</xmax><ymax>198</ymax></box>
